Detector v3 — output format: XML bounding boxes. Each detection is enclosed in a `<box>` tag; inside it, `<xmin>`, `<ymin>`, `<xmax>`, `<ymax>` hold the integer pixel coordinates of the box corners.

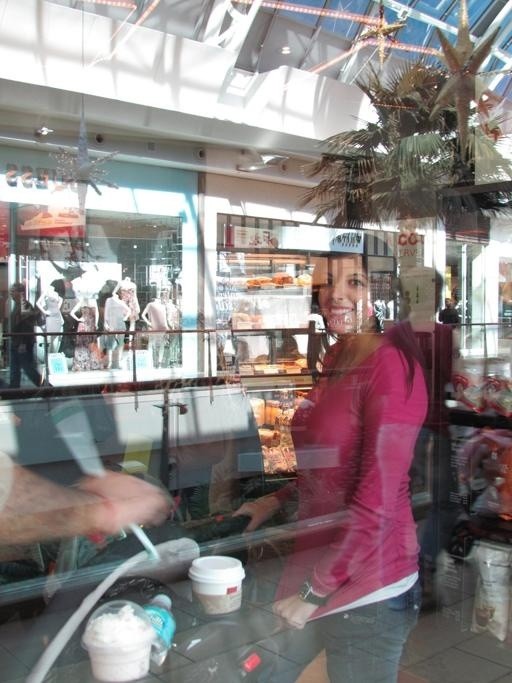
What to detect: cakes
<box><xmin>80</xmin><ymin>598</ymin><xmax>155</xmax><ymax>681</ymax></box>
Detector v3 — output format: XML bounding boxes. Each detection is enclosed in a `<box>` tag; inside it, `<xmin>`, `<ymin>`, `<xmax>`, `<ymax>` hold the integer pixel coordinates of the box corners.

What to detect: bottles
<box><xmin>139</xmin><ymin>591</ymin><xmax>176</xmax><ymax>667</ymax></box>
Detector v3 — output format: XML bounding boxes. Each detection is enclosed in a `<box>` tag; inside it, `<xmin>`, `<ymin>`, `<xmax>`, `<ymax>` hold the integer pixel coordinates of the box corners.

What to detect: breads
<box><xmin>245</xmin><ymin>272</ymin><xmax>314</xmax><ymax>290</ymax></box>
<box><xmin>234</xmin><ymin>310</ymin><xmax>265</xmax><ymax>330</ymax></box>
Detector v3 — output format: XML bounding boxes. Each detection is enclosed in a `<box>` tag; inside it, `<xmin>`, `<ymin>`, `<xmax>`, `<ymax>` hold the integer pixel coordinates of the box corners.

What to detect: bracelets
<box><xmin>79</xmin><ymin>491</ymin><xmax>119</xmax><ymax>546</ymax></box>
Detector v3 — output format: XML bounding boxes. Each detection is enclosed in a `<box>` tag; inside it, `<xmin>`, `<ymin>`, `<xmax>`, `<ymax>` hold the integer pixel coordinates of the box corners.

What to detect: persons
<box><xmin>231</xmin><ymin>234</ymin><xmax>430</xmax><ymax>683</ymax></box>
<box><xmin>8</xmin><ymin>280</ymin><xmax>182</xmax><ymax>387</ymax></box>
<box><xmin>384</xmin><ymin>265</ymin><xmax>461</xmax><ymax>611</ymax></box>
<box><xmin>35</xmin><ymin>224</ymin><xmax>121</xmax><ymax>298</ymax></box>
<box><xmin>0</xmin><ymin>451</ymin><xmax>169</xmax><ymax>546</ymax></box>
<box><xmin>438</xmin><ymin>297</ymin><xmax>462</xmax><ymax>329</ymax></box>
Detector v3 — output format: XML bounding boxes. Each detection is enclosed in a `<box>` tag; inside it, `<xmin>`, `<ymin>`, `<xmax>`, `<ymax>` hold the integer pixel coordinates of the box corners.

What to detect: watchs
<box><xmin>298</xmin><ymin>581</ymin><xmax>329</xmax><ymax>609</ymax></box>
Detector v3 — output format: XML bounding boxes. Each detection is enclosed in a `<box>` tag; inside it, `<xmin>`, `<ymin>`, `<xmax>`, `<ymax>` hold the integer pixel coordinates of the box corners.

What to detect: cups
<box><xmin>82</xmin><ymin>619</ymin><xmax>158</xmax><ymax>682</ymax></box>
<box><xmin>187</xmin><ymin>555</ymin><xmax>250</xmax><ymax>616</ymax></box>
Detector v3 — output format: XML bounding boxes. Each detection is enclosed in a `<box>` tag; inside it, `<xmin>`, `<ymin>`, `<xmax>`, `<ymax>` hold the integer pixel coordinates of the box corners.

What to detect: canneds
<box><xmin>265</xmin><ymin>400</ymin><xmax>281</xmax><ymax>429</ymax></box>
